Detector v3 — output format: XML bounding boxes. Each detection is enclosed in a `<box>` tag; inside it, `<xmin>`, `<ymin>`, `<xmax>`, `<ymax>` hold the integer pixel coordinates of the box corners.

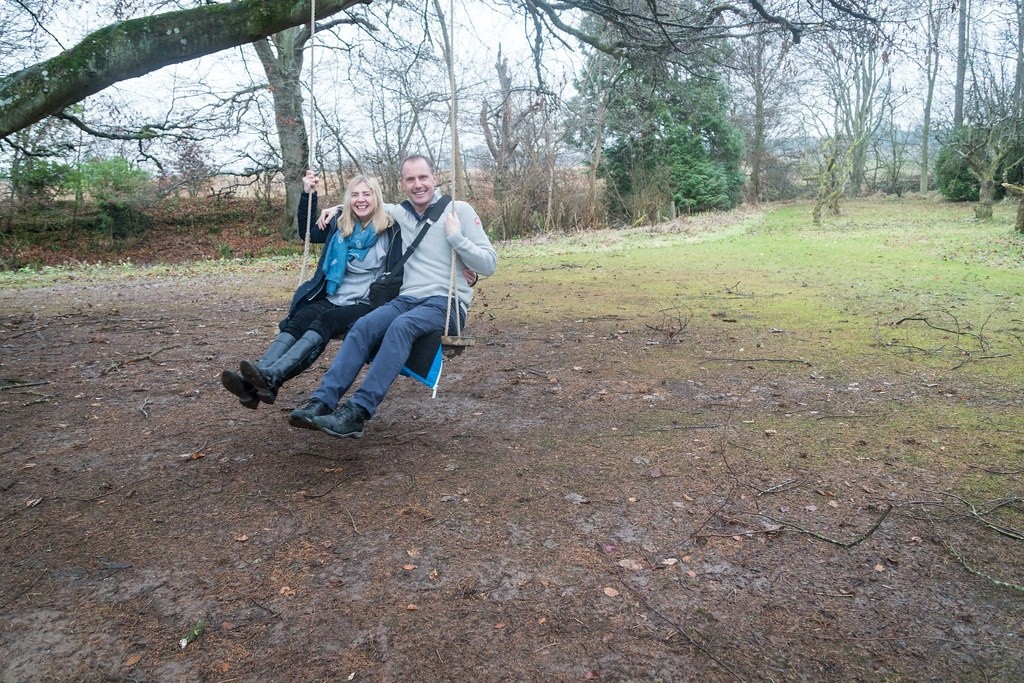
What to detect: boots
<box><xmin>240</xmin><ymin>329</ymin><xmax>325</xmax><ymax>405</ymax></box>
<box><xmin>220</xmin><ymin>332</ymin><xmax>297</xmax><ymax>409</ymax></box>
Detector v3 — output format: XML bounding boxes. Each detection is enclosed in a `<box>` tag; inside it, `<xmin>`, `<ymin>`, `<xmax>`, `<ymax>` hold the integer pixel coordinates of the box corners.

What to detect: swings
<box><xmin>299</xmin><ymin>0</ymin><xmax>476</xmax><ymax>358</ymax></box>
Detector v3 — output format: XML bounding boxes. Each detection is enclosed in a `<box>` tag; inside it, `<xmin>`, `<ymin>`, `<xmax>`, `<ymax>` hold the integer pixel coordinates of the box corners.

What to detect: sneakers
<box><xmin>313</xmin><ymin>397</ymin><xmax>364</xmax><ymax>439</ymax></box>
<box><xmin>288</xmin><ymin>398</ymin><xmax>335</xmax><ymax>431</ymax></box>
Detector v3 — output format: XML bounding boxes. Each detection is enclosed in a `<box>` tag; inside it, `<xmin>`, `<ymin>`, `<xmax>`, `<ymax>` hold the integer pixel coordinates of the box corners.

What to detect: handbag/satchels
<box><xmin>368</xmin><ymin>272</ymin><xmax>403</xmax><ymax>309</ymax></box>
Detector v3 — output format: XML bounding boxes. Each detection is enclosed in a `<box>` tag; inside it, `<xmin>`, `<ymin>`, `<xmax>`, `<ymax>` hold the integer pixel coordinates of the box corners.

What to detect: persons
<box><xmin>289</xmin><ymin>154</ymin><xmax>497</xmax><ymax>439</ymax></box>
<box><xmin>220</xmin><ymin>170</ymin><xmax>478</xmax><ymax>410</ymax></box>
